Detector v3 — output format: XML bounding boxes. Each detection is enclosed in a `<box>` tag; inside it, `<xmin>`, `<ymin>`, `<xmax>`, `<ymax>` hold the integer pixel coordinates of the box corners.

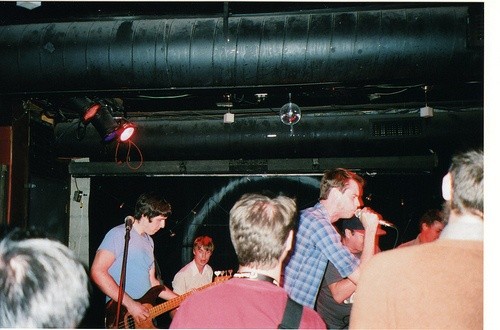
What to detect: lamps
<box><xmin>91</xmin><ymin>100</ymin><xmax>121</xmax><ymax>144</ymax></box>
<box><xmin>69</xmin><ymin>95</ymin><xmax>105</xmax><ymax>127</ymax></box>
<box><xmin>110</xmin><ymin>110</ymin><xmax>136</xmax><ymax>142</ymax></box>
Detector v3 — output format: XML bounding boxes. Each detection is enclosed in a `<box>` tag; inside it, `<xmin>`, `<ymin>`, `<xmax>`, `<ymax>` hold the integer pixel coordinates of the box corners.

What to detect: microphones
<box><xmin>354</xmin><ymin>209</ymin><xmax>394</xmax><ymax>228</ymax></box>
<box><xmin>124</xmin><ymin>216</ymin><xmax>135</xmax><ymax>231</ymax></box>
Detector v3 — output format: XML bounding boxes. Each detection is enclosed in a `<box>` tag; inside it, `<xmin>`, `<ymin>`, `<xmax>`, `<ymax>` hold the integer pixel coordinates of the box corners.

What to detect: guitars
<box><xmin>104</xmin><ymin>270</ymin><xmax>236</xmax><ymax>329</ymax></box>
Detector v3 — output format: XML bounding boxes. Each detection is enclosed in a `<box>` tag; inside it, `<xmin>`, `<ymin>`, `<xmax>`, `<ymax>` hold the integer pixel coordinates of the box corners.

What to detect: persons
<box><xmin>169</xmin><ymin>236</ymin><xmax>213</xmax><ymax>318</ymax></box>
<box><xmin>397</xmin><ymin>210</ymin><xmax>445</xmax><ymax>247</ymax></box>
<box><xmin>0</xmin><ymin>227</ymin><xmax>90</xmax><ymax>328</ymax></box>
<box><xmin>91</xmin><ymin>193</ymin><xmax>176</xmax><ymax>329</ymax></box>
<box><xmin>315</xmin><ymin>216</ymin><xmax>386</xmax><ymax>330</ymax></box>
<box><xmin>281</xmin><ymin>168</ymin><xmax>381</xmax><ymax>309</ymax></box>
<box><xmin>169</xmin><ymin>193</ymin><xmax>326</xmax><ymax>330</ymax></box>
<box><xmin>349</xmin><ymin>151</ymin><xmax>483</xmax><ymax>330</ymax></box>
